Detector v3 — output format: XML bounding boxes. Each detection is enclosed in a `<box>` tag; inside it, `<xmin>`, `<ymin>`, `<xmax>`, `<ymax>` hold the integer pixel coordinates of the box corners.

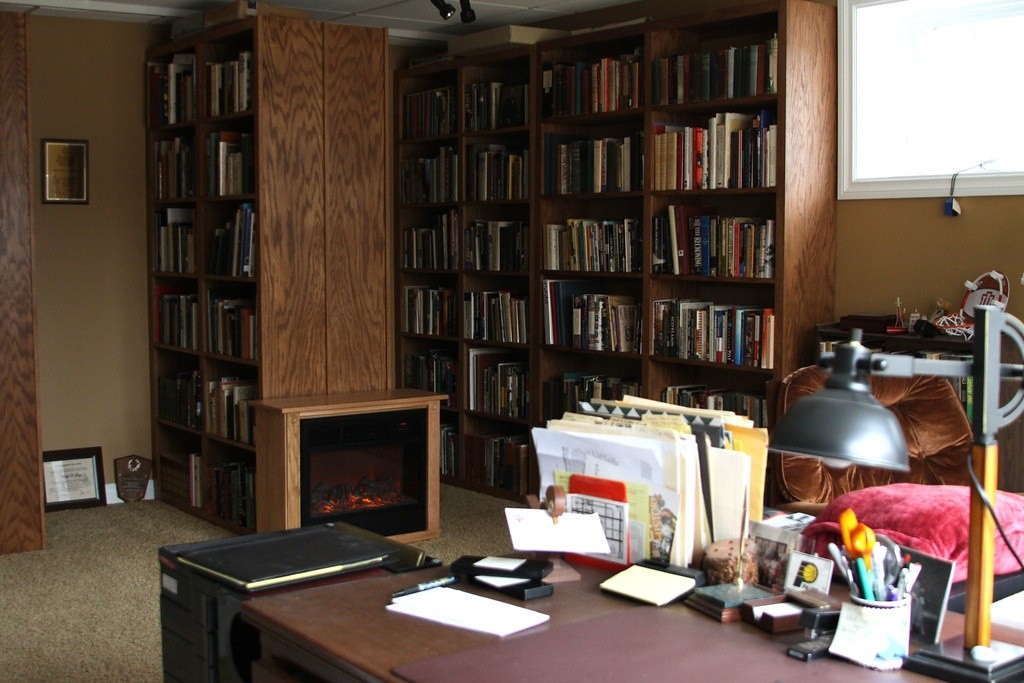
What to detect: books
<box><xmin>399</xmin><ymin>35</ymin><xmax>780</xmax><ymax>501</ymax></box>
<box><xmin>528</xmin><ymin>393</ymin><xmax>767</xmax><ymax>576</ymax></box>
<box><xmin>151</xmin><ymin>52</ymin><xmax>258</xmax><ymax>533</ymax></box>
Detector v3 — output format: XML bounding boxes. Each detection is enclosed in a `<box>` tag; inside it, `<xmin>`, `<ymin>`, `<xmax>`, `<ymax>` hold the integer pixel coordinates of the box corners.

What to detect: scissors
<box><xmin>840</xmin><ymin>508</ymin><xmax>875</xmax><ymax>570</ymax></box>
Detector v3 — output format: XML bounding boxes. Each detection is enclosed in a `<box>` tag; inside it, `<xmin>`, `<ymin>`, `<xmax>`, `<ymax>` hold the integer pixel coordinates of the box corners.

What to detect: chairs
<box><xmin>774</xmin><ymin>365</ymin><xmax>1024</xmax><ymax>584</ymax></box>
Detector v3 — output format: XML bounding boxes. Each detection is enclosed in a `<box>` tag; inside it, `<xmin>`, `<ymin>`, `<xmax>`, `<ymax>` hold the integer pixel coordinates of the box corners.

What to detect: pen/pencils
<box><xmin>829</xmin><ymin>542</ymin><xmax>922</xmax><ymax>603</ymax></box>
<box><xmin>392</xmin><ymin>574</ymin><xmax>454</xmax><ymax>598</ymax></box>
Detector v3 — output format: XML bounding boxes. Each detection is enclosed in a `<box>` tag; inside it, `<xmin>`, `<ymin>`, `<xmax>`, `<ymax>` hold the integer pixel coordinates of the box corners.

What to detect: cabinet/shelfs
<box><xmin>145</xmin><ymin>0</ymin><xmax>973</xmax><ymax>536</ymax></box>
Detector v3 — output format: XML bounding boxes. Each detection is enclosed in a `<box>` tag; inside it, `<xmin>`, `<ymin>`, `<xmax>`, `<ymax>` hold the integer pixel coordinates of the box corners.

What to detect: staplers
<box><xmin>787</xmin><ymin>607</ymin><xmax>841</xmax><ymax>662</ymax></box>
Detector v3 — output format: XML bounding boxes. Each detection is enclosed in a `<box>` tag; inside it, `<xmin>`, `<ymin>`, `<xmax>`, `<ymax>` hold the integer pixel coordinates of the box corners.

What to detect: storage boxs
<box><xmin>170</xmin><ymin>10</ymin><xmax>210</xmax><ymax>37</ymax></box>
<box><xmin>204</xmin><ymin>0</ymin><xmax>312</xmax><ymax>29</ymax></box>
<box><xmin>448</xmin><ymin>24</ymin><xmax>569</xmax><ymax>56</ymax></box>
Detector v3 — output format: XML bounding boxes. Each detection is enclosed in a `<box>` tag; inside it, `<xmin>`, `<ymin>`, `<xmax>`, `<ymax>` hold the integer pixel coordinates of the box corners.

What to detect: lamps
<box><xmin>460</xmin><ymin>0</ymin><xmax>477</xmax><ymax>23</ymax></box>
<box><xmin>430</xmin><ymin>0</ymin><xmax>455</xmax><ymax>20</ymax></box>
<box><xmin>767</xmin><ymin>304</ymin><xmax>1024</xmax><ymax>683</ymax></box>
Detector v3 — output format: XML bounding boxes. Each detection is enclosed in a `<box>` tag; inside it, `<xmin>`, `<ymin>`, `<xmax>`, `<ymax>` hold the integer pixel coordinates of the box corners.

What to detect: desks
<box><xmin>241</xmin><ymin>551</ymin><xmax>1024</xmax><ymax>683</ymax></box>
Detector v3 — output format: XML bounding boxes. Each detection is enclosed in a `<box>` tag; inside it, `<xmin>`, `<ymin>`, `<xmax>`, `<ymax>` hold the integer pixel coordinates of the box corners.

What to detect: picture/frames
<box><xmin>40</xmin><ymin>137</ymin><xmax>90</xmax><ymax>204</ymax></box>
